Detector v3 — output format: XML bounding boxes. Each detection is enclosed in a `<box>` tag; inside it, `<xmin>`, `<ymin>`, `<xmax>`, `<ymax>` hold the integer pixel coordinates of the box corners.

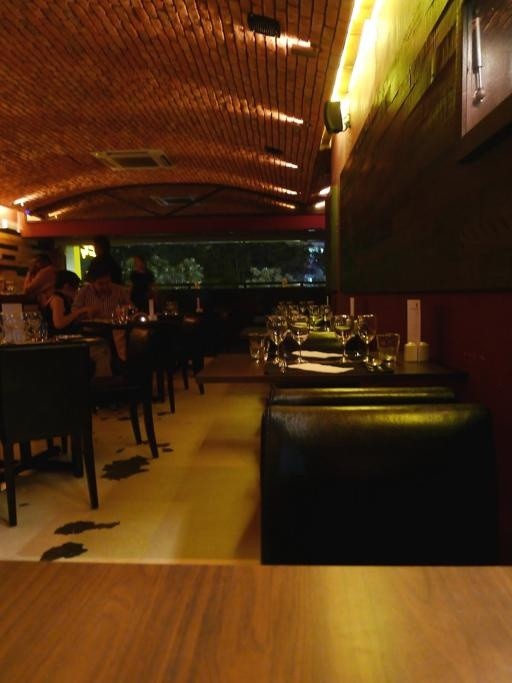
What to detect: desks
<box><xmin>193</xmin><ymin>323</ymin><xmax>468</xmax><ymax>403</ymax></box>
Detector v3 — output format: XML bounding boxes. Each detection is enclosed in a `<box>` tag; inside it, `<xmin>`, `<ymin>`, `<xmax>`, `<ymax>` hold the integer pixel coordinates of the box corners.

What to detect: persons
<box><xmin>71</xmin><ymin>260</ymin><xmax>135</xmax><ymax>322</ymax></box>
<box><xmin>127</xmin><ymin>252</ymin><xmax>160</xmax><ymax>313</ymax></box>
<box><xmin>87</xmin><ymin>233</ymin><xmax>123</xmax><ymax>285</ymax></box>
<box><xmin>22</xmin><ymin>251</ymin><xmax>57</xmax><ymax>330</ymax></box>
<box><xmin>41</xmin><ymin>268</ymin><xmax>102</xmax><ymax>340</ymax></box>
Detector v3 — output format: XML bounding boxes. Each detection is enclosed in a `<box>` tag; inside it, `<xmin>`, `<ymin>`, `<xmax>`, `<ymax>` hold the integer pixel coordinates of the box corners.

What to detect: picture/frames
<box><xmin>456</xmin><ymin>0</ymin><xmax>512</xmax><ymax>161</ymax></box>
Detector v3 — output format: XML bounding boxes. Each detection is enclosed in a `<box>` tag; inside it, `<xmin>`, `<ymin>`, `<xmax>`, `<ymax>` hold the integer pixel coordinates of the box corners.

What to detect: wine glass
<box><xmin>289</xmin><ymin>321</ymin><xmax>310</xmax><ymax>363</ymax></box>
<box><xmin>357</xmin><ymin>313</ymin><xmax>378</xmax><ymax>363</ymax></box>
<box><xmin>266</xmin><ymin>315</ymin><xmax>288</xmax><ymax>365</ymax></box>
<box><xmin>332</xmin><ymin>314</ymin><xmax>356</xmax><ymax>364</ymax></box>
<box><xmin>278</xmin><ymin>301</ymin><xmax>332</xmax><ymax>332</ymax></box>
<box><xmin>0</xmin><ymin>311</ymin><xmax>43</xmax><ymax>344</ymax></box>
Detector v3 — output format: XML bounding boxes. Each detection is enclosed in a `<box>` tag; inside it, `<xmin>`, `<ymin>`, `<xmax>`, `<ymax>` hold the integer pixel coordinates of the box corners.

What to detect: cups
<box><xmin>376</xmin><ymin>333</ymin><xmax>400</xmax><ymax>363</ymax></box>
